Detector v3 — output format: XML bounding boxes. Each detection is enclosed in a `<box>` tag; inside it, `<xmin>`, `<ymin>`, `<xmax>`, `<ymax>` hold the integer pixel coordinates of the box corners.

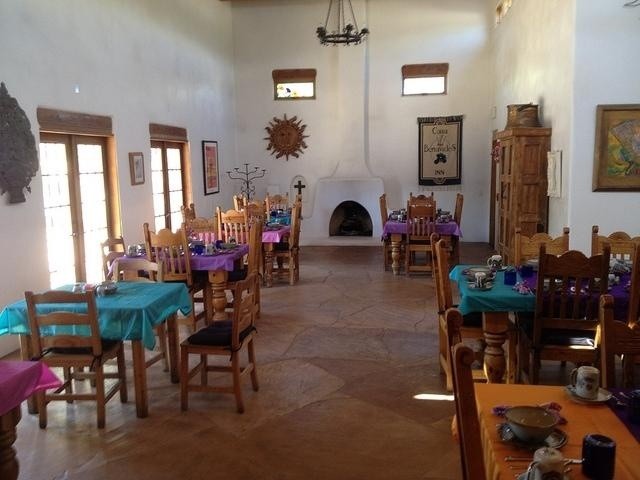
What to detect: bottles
<box><xmin>264</xmin><ymin>207</ymin><xmax>293</xmax><ymax>231</ymax></box>
<box><xmin>74</xmin><ymin>281</ymin><xmax>117</xmax><ymax>298</ymax></box>
<box><xmin>608</xmin><ymin>257</ymin><xmax>633</xmax><ymax>289</ymax></box>
<box><xmin>128</xmin><ymin>238</ymin><xmax>236</xmax><ymax>256</ymax></box>
<box><xmin>391</xmin><ymin>210</ymin><xmax>449</xmax><ymax>224</ymax></box>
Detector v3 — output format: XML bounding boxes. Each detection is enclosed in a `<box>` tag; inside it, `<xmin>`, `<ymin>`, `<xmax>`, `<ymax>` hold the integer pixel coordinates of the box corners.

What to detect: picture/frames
<box><xmin>202</xmin><ymin>139</ymin><xmax>220</xmax><ymax>197</ymax></box>
<box><xmin>417</xmin><ymin>114</ymin><xmax>463</xmax><ymax>186</ymax></box>
<box><xmin>591</xmin><ymin>104</ymin><xmax>640</xmax><ymax>191</ymax></box>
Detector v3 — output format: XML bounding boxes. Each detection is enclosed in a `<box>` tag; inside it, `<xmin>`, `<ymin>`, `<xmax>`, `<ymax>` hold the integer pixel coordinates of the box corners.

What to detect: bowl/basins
<box><xmin>504</xmin><ymin>407</ymin><xmax>560</xmax><ymax>440</ymax></box>
<box><xmin>469</xmin><ymin>266</ymin><xmax>490</xmax><ymax>278</ymax></box>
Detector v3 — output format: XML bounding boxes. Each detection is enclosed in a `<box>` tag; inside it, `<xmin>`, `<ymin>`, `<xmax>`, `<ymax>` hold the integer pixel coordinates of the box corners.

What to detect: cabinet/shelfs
<box><xmin>486</xmin><ymin>102</ymin><xmax>554</xmax><ymax>272</ymax></box>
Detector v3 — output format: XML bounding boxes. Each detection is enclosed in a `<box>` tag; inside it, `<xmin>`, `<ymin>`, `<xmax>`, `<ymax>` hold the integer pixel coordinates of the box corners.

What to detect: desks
<box><xmin>1</xmin><ymin>361</ymin><xmax>40</xmax><ymax>480</ymax></box>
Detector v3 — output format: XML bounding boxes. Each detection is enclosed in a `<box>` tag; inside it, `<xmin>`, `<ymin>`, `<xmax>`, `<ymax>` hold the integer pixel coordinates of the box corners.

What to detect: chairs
<box><xmin>597</xmin><ymin>291</ymin><xmax>638</xmax><ymax>430</ymax></box>
<box><xmin>431</xmin><ymin>225</ymin><xmax>639</xmax><ymax>390</ymax></box>
<box><xmin>410</xmin><ymin>191</ymin><xmax>435</xmax><ymax>200</ymax></box>
<box><xmin>448</xmin><ymin>341</ymin><xmax>486</xmax><ymax>479</ymax></box>
<box><xmin>404</xmin><ymin>200</ymin><xmax>437</xmax><ymax>277</ymax></box>
<box><xmin>448</xmin><ymin>193</ymin><xmax>464</xmax><ymax>267</ymax></box>
<box><xmin>179</xmin><ymin>269</ymin><xmax>260</xmax><ymax>415</ymax></box>
<box><xmin>23</xmin><ymin>290</ymin><xmax>128</xmax><ymax>431</ymax></box>
<box><xmin>380</xmin><ymin>193</ymin><xmax>405</xmax><ymax>272</ymax></box>
<box><xmin>101</xmin><ymin>194</ymin><xmax>303</xmax><ymax>331</ymax></box>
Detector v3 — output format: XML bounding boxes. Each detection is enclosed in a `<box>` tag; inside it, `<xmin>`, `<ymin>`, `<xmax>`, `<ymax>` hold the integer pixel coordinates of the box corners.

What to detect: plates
<box><xmin>467</xmin><ymin>284</ymin><xmax>493</xmax><ymax>290</ymax></box>
<box><xmin>564</xmin><ymin>385</ymin><xmax>614</xmax><ymax>407</ymax></box>
<box><xmin>516</xmin><ymin>472</ymin><xmax>527</xmax><ymax>480</ymax></box>
<box><xmin>495</xmin><ymin>421</ymin><xmax>569</xmax><ymax>449</ymax></box>
<box><xmin>485</xmin><ymin>265</ymin><xmax>508</xmax><ymax>271</ymax></box>
<box><xmin>465</xmin><ymin>274</ymin><xmax>495</xmax><ymax>281</ymax></box>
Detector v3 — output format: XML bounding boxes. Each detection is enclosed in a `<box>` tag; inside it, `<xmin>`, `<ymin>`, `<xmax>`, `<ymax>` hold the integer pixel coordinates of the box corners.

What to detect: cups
<box><xmin>475</xmin><ymin>273</ymin><xmax>488</xmax><ymax>288</ymax></box>
<box><xmin>488</xmin><ymin>255</ymin><xmax>503</xmax><ymax>271</ymax></box>
<box><xmin>582</xmin><ymin>435</ymin><xmax>617</xmax><ymax>477</ymax></box>
<box><xmin>505</xmin><ymin>271</ymin><xmax>518</xmax><ymax>286</ymax></box>
<box><xmin>527</xmin><ymin>446</ymin><xmax>564</xmax><ymax>480</ymax></box>
<box><xmin>569</xmin><ymin>364</ymin><xmax>599</xmax><ymax>399</ymax></box>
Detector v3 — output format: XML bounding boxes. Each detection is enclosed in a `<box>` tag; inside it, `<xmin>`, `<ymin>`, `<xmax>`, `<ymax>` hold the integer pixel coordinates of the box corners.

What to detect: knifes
<box><xmin>504</xmin><ymin>453</ymin><xmax>583</xmax><ymax>474</ymax></box>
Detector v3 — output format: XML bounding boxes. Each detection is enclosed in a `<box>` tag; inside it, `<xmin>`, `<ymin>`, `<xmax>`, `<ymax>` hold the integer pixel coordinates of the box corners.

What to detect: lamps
<box><xmin>312</xmin><ymin>0</ymin><xmax>374</xmax><ymax>47</ymax></box>
